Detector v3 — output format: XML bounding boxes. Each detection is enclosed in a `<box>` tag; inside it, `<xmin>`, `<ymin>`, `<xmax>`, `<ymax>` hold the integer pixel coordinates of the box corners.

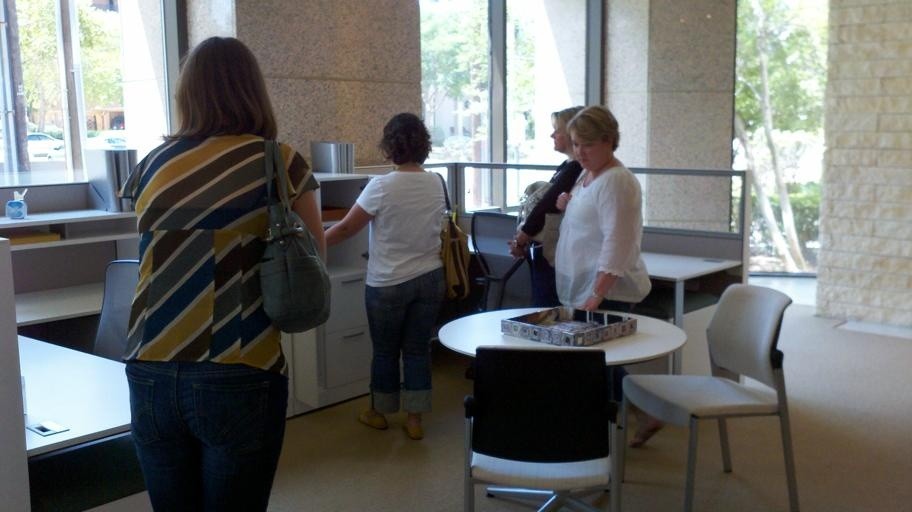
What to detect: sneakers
<box><xmin>629</xmin><ymin>420</ymin><xmax>662</xmax><ymax>445</ymax></box>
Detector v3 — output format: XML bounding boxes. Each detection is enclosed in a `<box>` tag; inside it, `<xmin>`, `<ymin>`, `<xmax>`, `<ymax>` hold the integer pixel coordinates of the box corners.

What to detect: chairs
<box><xmin>619</xmin><ymin>284</ymin><xmax>799</xmax><ymax>512</ymax></box>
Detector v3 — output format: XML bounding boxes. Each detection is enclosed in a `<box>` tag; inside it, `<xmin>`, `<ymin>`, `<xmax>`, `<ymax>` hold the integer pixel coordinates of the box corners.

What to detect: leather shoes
<box><xmin>403</xmin><ymin>416</ymin><xmax>425</xmax><ymax>440</ymax></box>
<box><xmin>359</xmin><ymin>408</ymin><xmax>388</xmax><ymax>430</ymax></box>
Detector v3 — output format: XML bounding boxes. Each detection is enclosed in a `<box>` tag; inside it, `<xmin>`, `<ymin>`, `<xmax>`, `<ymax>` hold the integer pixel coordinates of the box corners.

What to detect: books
<box><xmin>84</xmin><ymin>149</ymin><xmax>122</xmax><ymax>213</ymax></box>
<box><xmin>310</xmin><ymin>140</ymin><xmax>357</xmax><ymax>174</ymax></box>
<box><xmin>106</xmin><ymin>148</ymin><xmax>130</xmax><ymax>213</ymax></box>
<box><xmin>2</xmin><ymin>229</ymin><xmax>62</xmax><ymax>244</ymax></box>
<box><xmin>320</xmin><ymin>204</ymin><xmax>352</xmax><ymax>223</ymax></box>
<box><xmin>124</xmin><ymin>148</ymin><xmax>137</xmax><ymax>173</ymax></box>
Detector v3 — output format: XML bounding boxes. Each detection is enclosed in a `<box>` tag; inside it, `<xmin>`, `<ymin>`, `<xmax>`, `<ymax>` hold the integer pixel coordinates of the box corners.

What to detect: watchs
<box><xmin>512</xmin><ymin>238</ymin><xmax>521</xmax><ymax>248</ymax></box>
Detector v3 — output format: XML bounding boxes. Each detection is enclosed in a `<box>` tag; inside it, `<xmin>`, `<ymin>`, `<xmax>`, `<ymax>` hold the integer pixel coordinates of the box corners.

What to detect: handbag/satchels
<box><xmin>260</xmin><ymin>204</ymin><xmax>331</xmax><ymax>333</ymax></box>
<box><xmin>439</xmin><ymin>215</ymin><xmax>471</xmax><ymax>300</ymax></box>
<box><xmin>516</xmin><ymin>181</ymin><xmax>565</xmax><ymax>269</ymax></box>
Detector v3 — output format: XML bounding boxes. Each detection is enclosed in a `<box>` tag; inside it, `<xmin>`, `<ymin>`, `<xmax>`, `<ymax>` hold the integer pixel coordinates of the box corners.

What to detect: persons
<box><xmin>553</xmin><ymin>105</ymin><xmax>667</xmax><ymax>449</ymax></box>
<box><xmin>117</xmin><ymin>36</ymin><xmax>330</xmax><ymax>511</ymax></box>
<box><xmin>506</xmin><ymin>103</ymin><xmax>585</xmax><ymax>305</ymax></box>
<box><xmin>319</xmin><ymin>111</ymin><xmax>453</xmax><ymax>440</ymax></box>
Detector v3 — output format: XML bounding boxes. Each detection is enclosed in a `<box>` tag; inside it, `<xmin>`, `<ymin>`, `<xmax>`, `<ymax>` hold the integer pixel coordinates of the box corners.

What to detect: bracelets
<box><xmin>590</xmin><ymin>290</ymin><xmax>604</xmax><ymax>300</ymax></box>
